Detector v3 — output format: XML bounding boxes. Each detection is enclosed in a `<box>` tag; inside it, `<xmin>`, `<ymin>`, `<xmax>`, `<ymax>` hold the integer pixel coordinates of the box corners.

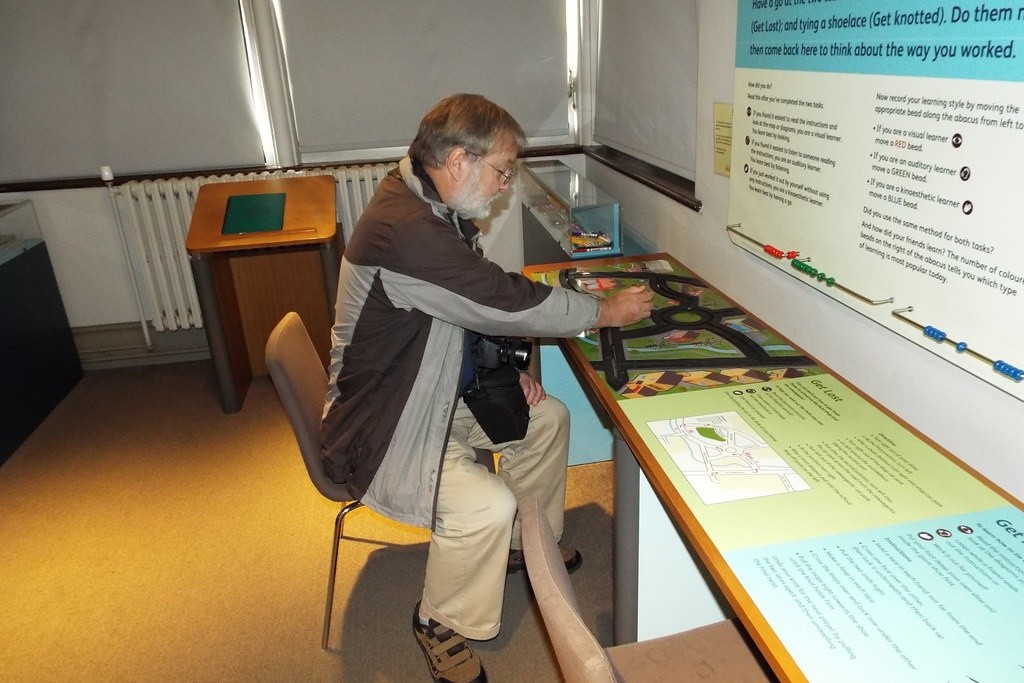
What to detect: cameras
<box><xmin>477</xmin><ymin>334</ymin><xmax>533</xmax><ymax>370</ymax></box>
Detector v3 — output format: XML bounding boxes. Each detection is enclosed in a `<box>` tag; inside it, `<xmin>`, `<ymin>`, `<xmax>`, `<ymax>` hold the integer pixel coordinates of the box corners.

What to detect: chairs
<box><xmin>262</xmin><ymin>310</ymin><xmax>491</xmax><ymax>652</ymax></box>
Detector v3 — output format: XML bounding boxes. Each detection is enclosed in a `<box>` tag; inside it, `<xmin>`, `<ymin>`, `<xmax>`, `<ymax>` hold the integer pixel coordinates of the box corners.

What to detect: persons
<box><xmin>317</xmin><ymin>93</ymin><xmax>655</xmax><ymax>683</ymax></box>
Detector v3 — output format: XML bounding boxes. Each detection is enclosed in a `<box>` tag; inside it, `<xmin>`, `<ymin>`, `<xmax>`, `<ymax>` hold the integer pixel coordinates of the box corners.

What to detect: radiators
<box><xmin>98</xmin><ymin>158</ymin><xmax>408</xmax><ymax>356</ymax></box>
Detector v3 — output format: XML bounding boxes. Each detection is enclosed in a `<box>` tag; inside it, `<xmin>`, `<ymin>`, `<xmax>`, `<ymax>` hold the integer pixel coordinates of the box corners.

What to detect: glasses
<box><xmin>465</xmin><ymin>150</ymin><xmax>514</xmax><ymax>185</ymax></box>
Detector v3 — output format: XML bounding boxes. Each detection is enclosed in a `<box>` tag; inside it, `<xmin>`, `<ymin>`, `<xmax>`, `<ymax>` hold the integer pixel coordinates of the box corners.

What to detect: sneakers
<box><xmin>507</xmin><ymin>545</ymin><xmax>583</xmax><ymax>574</ymax></box>
<box><xmin>412</xmin><ymin>599</ymin><xmax>487</xmax><ymax>683</ymax></box>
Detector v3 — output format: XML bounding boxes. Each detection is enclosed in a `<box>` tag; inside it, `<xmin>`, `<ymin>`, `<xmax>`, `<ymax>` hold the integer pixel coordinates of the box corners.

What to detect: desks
<box><xmin>512</xmin><ymin>250</ymin><xmax>1023</xmax><ymax>683</ymax></box>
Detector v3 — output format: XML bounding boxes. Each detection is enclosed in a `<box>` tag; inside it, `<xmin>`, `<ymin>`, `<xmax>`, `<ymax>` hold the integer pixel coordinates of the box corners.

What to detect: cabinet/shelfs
<box><xmin>521</xmin><ymin>159</ymin><xmax>655</xmax><ymax>270</ymax></box>
<box><xmin>1</xmin><ymin>197</ymin><xmax>85</xmax><ymax>471</ymax></box>
<box><xmin>183</xmin><ymin>172</ymin><xmax>344</xmax><ymax>415</ymax></box>
